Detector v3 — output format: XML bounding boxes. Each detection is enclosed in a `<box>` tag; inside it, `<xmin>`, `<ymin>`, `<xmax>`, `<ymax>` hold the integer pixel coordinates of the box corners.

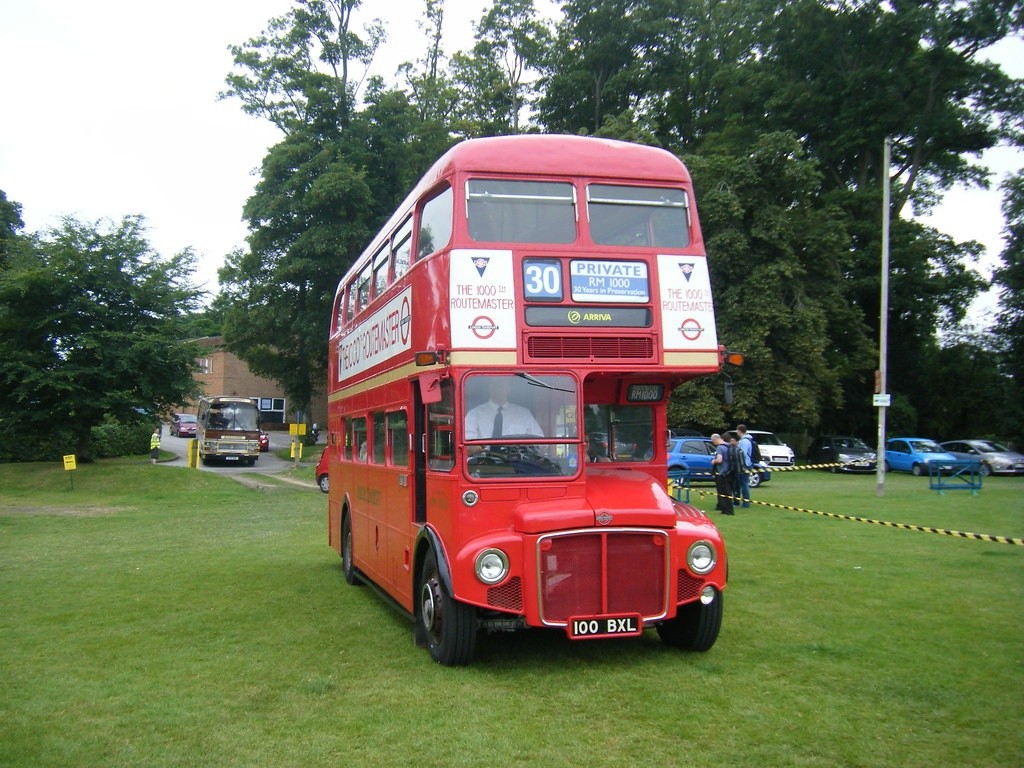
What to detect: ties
<box><xmin>490</xmin><ymin>407</ymin><xmax>503</xmax><ymax>451</ymax></box>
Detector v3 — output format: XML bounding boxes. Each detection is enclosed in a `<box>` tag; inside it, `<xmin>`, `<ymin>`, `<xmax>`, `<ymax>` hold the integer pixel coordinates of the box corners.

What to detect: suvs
<box><xmin>647</xmin><ymin>427</ymin><xmax>706</xmax><ymax>452</ymax></box>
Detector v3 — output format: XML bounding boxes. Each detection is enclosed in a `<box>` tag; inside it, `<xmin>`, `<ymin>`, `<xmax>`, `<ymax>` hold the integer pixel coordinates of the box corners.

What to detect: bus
<box><xmin>195</xmin><ymin>396</ymin><xmax>262</xmax><ymax>466</ymax></box>
<box><xmin>328</xmin><ymin>130</ymin><xmax>745</xmax><ymax>669</ymax></box>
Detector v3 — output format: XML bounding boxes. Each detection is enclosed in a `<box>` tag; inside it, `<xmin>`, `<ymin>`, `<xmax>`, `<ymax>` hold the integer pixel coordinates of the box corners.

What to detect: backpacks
<box><xmin>719</xmin><ymin>444</ymin><xmax>744</xmax><ymax>473</ymax></box>
<box><xmin>743</xmin><ymin>438</ymin><xmax>762</xmax><ymax>463</ymax></box>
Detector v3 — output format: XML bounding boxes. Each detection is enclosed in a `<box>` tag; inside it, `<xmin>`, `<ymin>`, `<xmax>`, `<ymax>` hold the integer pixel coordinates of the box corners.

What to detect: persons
<box><xmin>565</xmin><ymin>433</ymin><xmax>612</xmax><ymax>466</ymax></box>
<box><xmin>711</xmin><ymin>424</ymin><xmax>755</xmax><ymax>515</ymax></box>
<box><xmin>150</xmin><ymin>428</ymin><xmax>162</xmax><ymax>466</ymax></box>
<box><xmin>464</xmin><ymin>376</ymin><xmax>546</xmax><ymax>462</ymax></box>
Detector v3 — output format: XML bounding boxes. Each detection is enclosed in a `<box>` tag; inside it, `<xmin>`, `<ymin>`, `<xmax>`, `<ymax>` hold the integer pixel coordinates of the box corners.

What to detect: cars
<box><xmin>727</xmin><ymin>430</ymin><xmax>796</xmax><ymax>470</ymax></box>
<box><xmin>260</xmin><ymin>428</ymin><xmax>269</xmax><ymax>451</ymax></box>
<box><xmin>169</xmin><ymin>414</ymin><xmax>197</xmax><ymax>437</ymax></box>
<box><xmin>807</xmin><ymin>436</ymin><xmax>877</xmax><ymax>474</ymax></box>
<box><xmin>644</xmin><ymin>437</ymin><xmax>772</xmax><ymax>488</ymax></box>
<box><xmin>939</xmin><ymin>439</ymin><xmax>1024</xmax><ymax>477</ymax></box>
<box><xmin>885</xmin><ymin>438</ymin><xmax>959</xmax><ymax>477</ymax></box>
<box><xmin>587</xmin><ymin>432</ymin><xmax>639</xmax><ymax>462</ymax></box>
